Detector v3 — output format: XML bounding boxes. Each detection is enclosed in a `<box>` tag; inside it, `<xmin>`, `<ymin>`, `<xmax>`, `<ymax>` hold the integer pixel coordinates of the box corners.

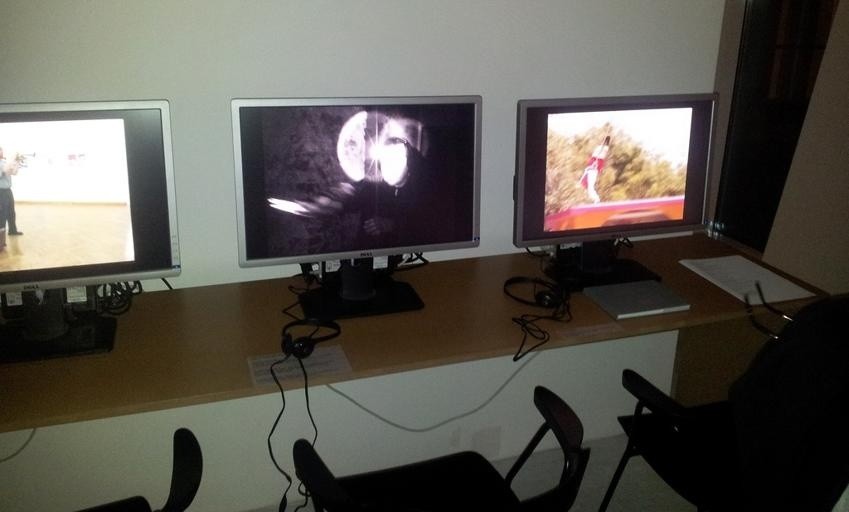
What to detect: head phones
<box><xmin>281</xmin><ymin>317</ymin><xmax>342</xmax><ymax>359</ymax></box>
<box><xmin>503</xmin><ymin>276</ymin><xmax>572</xmax><ymax>312</ymax></box>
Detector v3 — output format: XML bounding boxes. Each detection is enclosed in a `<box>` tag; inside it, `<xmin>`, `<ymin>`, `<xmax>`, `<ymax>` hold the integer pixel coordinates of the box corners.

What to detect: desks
<box><xmin>2</xmin><ymin>232</ymin><xmax>832</xmax><ymax>435</ymax></box>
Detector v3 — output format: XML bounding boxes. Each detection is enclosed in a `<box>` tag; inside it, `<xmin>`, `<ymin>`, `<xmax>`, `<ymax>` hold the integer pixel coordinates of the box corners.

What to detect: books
<box><xmin>679</xmin><ymin>252</ymin><xmax>815</xmax><ymax>308</ymax></box>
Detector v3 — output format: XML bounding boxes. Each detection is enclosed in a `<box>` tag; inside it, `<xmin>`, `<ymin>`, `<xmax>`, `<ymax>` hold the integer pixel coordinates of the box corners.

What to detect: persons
<box><xmin>0</xmin><ymin>146</ymin><xmax>26</xmax><ymax>242</ymax></box>
<box><xmin>584</xmin><ymin>136</ymin><xmax>610</xmax><ymax>205</ymax></box>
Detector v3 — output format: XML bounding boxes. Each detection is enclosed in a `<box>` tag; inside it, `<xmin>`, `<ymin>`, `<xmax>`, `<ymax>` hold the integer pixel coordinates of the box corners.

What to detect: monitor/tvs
<box><xmin>0</xmin><ymin>99</ymin><xmax>181</xmax><ymax>366</ymax></box>
<box><xmin>229</xmin><ymin>94</ymin><xmax>482</xmax><ymax>320</ymax></box>
<box><xmin>514</xmin><ymin>91</ymin><xmax>719</xmax><ymax>292</ymax></box>
